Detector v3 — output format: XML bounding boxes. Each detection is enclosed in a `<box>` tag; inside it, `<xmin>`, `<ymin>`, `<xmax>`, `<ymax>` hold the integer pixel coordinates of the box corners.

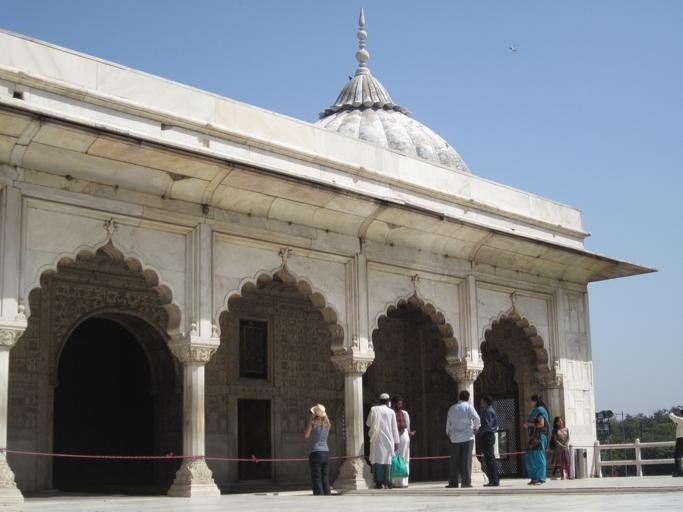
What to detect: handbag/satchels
<box><xmin>550</xmin><ymin>430</ymin><xmax>559</xmax><ymax>448</ymax></box>
<box><xmin>390</xmin><ymin>449</ymin><xmax>408</xmax><ymax>477</ymax></box>
<box><xmin>529</xmin><ymin>420</ymin><xmax>540</xmax><ymax>448</ymax></box>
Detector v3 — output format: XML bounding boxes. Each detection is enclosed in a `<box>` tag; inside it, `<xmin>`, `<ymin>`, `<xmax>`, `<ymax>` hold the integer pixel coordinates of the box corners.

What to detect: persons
<box><xmin>520</xmin><ymin>393</ymin><xmax>551</xmax><ymax>486</ymax></box>
<box><xmin>302</xmin><ymin>403</ymin><xmax>332</xmax><ymax>497</ymax></box>
<box><xmin>443</xmin><ymin>389</ymin><xmax>482</xmax><ymax>488</ymax></box>
<box><xmin>391</xmin><ymin>395</ymin><xmax>417</xmax><ymax>489</ymax></box>
<box><xmin>364</xmin><ymin>391</ymin><xmax>400</xmax><ymax>489</ymax></box>
<box><xmin>665</xmin><ymin>409</ymin><xmax>682</xmax><ymax>478</ymax></box>
<box><xmin>550</xmin><ymin>415</ymin><xmax>575</xmax><ymax>481</ymax></box>
<box><xmin>478</xmin><ymin>394</ymin><xmax>500</xmax><ymax>487</ymax></box>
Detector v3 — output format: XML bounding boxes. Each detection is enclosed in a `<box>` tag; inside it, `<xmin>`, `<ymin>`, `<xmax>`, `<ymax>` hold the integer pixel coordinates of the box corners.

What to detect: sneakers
<box><xmin>484</xmin><ymin>483</ymin><xmax>499</xmax><ymax>487</ymax></box>
<box><xmin>527</xmin><ymin>479</ymin><xmax>544</xmax><ymax>486</ymax></box>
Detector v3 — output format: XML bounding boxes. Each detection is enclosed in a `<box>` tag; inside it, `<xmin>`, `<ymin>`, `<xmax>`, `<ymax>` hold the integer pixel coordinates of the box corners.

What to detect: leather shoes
<box><xmin>445</xmin><ymin>484</ymin><xmax>458</xmax><ymax>488</ymax></box>
<box><xmin>461</xmin><ymin>484</ymin><xmax>472</xmax><ymax>488</ymax></box>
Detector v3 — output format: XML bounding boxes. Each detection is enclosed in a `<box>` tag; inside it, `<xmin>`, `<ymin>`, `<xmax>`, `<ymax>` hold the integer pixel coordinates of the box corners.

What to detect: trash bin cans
<box><xmin>574</xmin><ymin>448</ymin><xmax>588</xmax><ymax>479</ymax></box>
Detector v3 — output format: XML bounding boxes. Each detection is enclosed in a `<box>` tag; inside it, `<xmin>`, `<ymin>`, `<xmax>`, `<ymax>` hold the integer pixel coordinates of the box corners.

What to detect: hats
<box><xmin>310</xmin><ymin>404</ymin><xmax>326</xmax><ymax>418</ymax></box>
<box><xmin>379</xmin><ymin>393</ymin><xmax>389</xmax><ymax>400</ymax></box>
